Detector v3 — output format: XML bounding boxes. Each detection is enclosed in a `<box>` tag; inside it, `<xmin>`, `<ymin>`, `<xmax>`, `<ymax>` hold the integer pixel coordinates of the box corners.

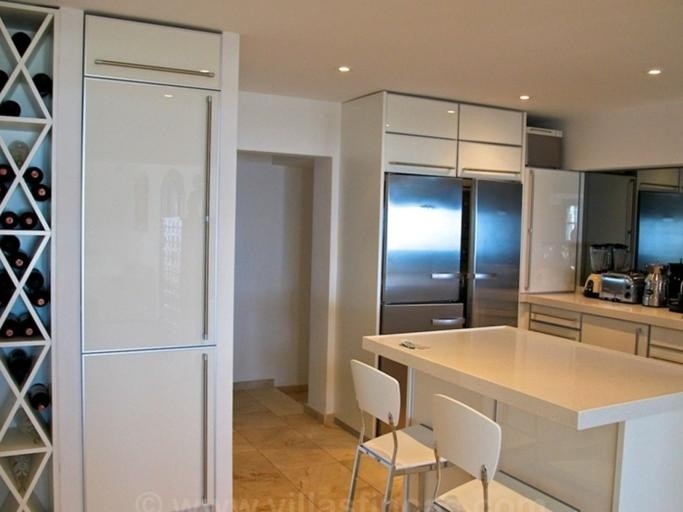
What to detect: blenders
<box><xmin>607</xmin><ymin>242</ymin><xmax>632</xmax><ymax>274</ymax></box>
<box><xmin>583</xmin><ymin>240</ymin><xmax>611</xmax><ymax>299</ymax></box>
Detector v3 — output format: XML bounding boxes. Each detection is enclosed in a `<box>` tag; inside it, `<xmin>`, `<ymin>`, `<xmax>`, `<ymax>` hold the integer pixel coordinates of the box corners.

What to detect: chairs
<box><xmin>346</xmin><ymin>358</ymin><xmax>453</xmax><ymax>512</ymax></box>
<box><xmin>423</xmin><ymin>392</ymin><xmax>553</xmax><ymax>512</ymax></box>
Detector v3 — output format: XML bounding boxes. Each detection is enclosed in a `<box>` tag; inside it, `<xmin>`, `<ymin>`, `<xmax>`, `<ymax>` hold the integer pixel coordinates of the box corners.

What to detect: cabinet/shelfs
<box><xmin>0</xmin><ymin>0</ymin><xmax>240</xmax><ymax>512</ymax></box>
<box><xmin>518</xmin><ymin>292</ymin><xmax>683</xmax><ymax>365</ymax></box>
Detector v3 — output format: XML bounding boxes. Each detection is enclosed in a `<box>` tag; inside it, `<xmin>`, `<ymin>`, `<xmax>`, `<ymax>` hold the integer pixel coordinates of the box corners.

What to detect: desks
<box><xmin>361</xmin><ymin>324</ymin><xmax>682</xmax><ymax>512</ymax></box>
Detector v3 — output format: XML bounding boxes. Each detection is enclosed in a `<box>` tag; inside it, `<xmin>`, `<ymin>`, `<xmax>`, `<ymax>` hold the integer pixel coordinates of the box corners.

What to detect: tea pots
<box><xmin>642</xmin><ymin>260</ymin><xmax>674</xmax><ymax>308</ymax></box>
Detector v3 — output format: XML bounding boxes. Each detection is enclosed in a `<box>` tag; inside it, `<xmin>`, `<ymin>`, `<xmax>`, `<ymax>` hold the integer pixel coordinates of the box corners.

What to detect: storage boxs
<box><xmin>526</xmin><ymin>127</ymin><xmax>565</xmax><ymax>170</ymax></box>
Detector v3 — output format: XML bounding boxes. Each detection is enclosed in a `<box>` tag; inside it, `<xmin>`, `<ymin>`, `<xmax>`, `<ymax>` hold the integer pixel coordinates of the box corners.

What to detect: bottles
<box><xmin>0</xmin><ymin>26</ymin><xmax>52</xmax><ymax>415</ymax></box>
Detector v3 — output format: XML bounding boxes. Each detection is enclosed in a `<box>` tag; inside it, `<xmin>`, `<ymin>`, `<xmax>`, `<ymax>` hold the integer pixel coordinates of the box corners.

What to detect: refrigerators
<box><xmin>373</xmin><ymin>166</ymin><xmax>527</xmax><ymax>439</ymax></box>
<box><xmin>638</xmin><ymin>189</ymin><xmax>683</xmax><ymax>268</ymax></box>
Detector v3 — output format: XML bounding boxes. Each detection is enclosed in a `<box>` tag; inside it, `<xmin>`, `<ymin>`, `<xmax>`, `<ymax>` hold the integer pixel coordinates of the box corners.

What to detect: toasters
<box><xmin>599</xmin><ymin>269</ymin><xmax>642</xmax><ymax>305</ymax></box>
